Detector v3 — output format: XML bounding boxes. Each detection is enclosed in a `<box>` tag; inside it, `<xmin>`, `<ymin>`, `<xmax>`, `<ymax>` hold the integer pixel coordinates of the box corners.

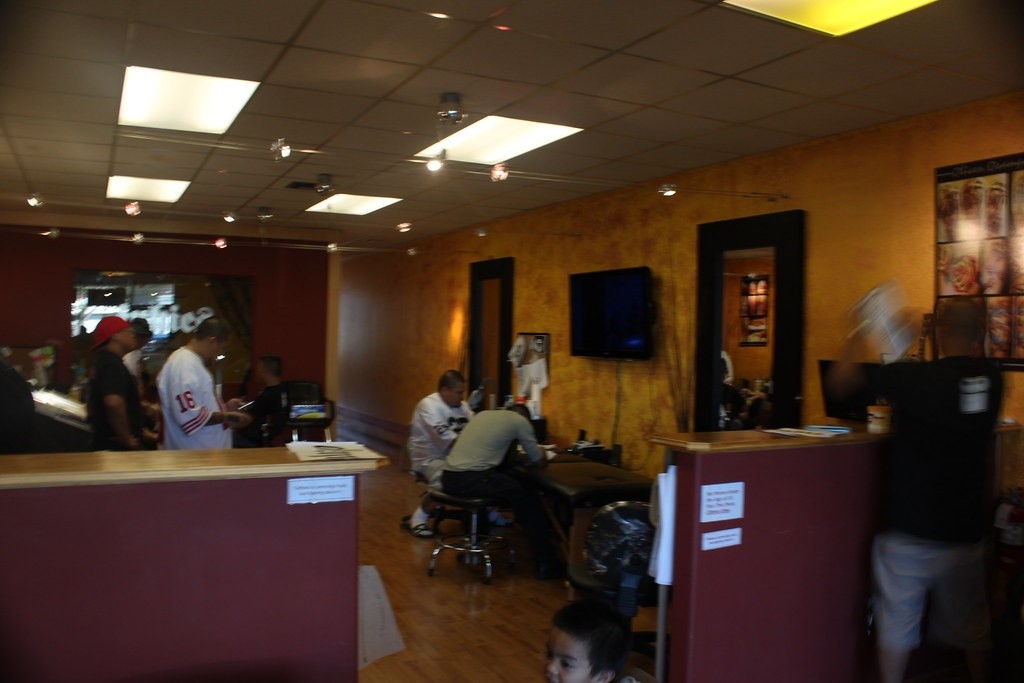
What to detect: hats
<box><xmin>88</xmin><ymin>315</ymin><xmax>130</xmax><ymax>352</ymax></box>
<box><xmin>126</xmin><ymin>318</ymin><xmax>155</xmax><ymax>338</ymax></box>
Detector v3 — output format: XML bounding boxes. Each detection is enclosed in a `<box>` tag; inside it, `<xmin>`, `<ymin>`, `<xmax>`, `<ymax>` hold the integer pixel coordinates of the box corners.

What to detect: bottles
<box><xmin>867</xmin><ymin>405</ymin><xmax>890</xmax><ymax>434</ymax></box>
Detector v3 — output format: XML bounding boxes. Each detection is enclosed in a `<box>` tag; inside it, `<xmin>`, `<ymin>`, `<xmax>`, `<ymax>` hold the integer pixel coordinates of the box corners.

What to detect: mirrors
<box><xmin>466</xmin><ymin>257</ymin><xmax>516</xmax><ymax>412</ymax></box>
<box><xmin>693</xmin><ymin>209</ymin><xmax>807</xmax><ymax>430</ymax></box>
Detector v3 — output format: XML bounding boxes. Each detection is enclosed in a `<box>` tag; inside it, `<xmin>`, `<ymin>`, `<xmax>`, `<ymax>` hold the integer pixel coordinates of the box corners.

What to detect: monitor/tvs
<box><xmin>569</xmin><ymin>266</ymin><xmax>654</xmax><ymax>360</ymax></box>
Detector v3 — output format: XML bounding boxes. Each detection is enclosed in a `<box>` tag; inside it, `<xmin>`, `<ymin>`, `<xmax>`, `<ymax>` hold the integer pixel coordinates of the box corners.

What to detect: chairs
<box><xmin>584</xmin><ymin>500</ymin><xmax>669</xmax><ymax>660</ymax></box>
<box><xmin>279</xmin><ymin>381</ymin><xmax>335</xmax><ymax>442</ymax></box>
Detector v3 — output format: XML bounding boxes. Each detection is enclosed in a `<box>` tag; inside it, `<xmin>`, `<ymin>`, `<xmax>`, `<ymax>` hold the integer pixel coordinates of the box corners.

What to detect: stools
<box><xmin>400</xmin><ymin>472</ymin><xmax>517</xmax><ymax>585</ymax></box>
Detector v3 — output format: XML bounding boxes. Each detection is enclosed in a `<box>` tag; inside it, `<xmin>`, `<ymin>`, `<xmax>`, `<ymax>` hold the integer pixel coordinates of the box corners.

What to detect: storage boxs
<box><xmin>819</xmin><ymin>359</ymin><xmax>914</xmax><ymax>421</ymax></box>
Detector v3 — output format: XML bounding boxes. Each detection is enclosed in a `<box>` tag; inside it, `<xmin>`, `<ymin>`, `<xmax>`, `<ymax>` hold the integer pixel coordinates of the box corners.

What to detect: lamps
<box><xmin>434</xmin><ymin>92</ymin><xmax>465</xmax><ymax>126</ymax></box>
<box><xmin>314</xmin><ymin>173</ymin><xmax>335</xmax><ymax>197</ymax></box>
<box><xmin>256</xmin><ymin>208</ymin><xmax>273</xmax><ymax>223</ymax></box>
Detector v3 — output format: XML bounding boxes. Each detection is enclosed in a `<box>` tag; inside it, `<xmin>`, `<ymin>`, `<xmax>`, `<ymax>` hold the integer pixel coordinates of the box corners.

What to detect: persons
<box><xmin>544</xmin><ymin>596</ymin><xmax>639</xmax><ymax>683</ymax></box>
<box><xmin>155</xmin><ymin>315</ymin><xmax>252</xmax><ymax>451</ymax></box>
<box><xmin>871</xmin><ymin>297</ymin><xmax>1003</xmax><ymax>683</ymax></box>
<box><xmin>407</xmin><ymin>369</ymin><xmax>515</xmax><ymax>539</ymax></box>
<box><xmin>166</xmin><ymin>308</ymin><xmax>291</xmax><ymax>449</ymax></box>
<box><xmin>719</xmin><ymin>357</ymin><xmax>774</xmax><ymax>428</ymax></box>
<box><xmin>446</xmin><ymin>404</ymin><xmax>571</xmax><ymax>580</ymax></box>
<box><xmin>0</xmin><ymin>362</ymin><xmax>35</xmax><ymax>454</ymax></box>
<box><xmin>77</xmin><ymin>316</ymin><xmax>159</xmax><ymax>451</ymax></box>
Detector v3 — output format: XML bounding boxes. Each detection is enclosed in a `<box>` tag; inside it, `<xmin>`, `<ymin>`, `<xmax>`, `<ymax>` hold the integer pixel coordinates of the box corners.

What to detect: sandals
<box><xmin>399</xmin><ymin>516</ymin><xmax>435</xmax><ymax>540</ymax></box>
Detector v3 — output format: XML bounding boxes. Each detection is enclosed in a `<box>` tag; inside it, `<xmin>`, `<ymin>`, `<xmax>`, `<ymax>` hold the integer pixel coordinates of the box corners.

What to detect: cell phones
<box><xmin>238</xmin><ymin>401</ymin><xmax>255</xmax><ymax>410</ymax></box>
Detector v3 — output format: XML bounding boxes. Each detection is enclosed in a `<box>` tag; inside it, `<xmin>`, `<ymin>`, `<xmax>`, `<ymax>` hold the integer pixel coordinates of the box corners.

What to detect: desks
<box><xmin>515</xmin><ymin>452</ymin><xmax>657</xmax><ymax>580</ymax></box>
